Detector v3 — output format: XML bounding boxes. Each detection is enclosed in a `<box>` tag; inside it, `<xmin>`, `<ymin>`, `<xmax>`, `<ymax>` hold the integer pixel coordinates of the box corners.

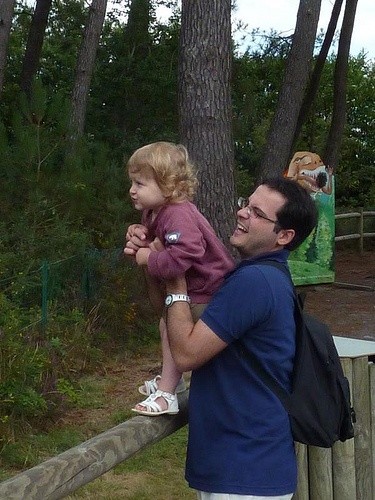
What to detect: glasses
<box><xmin>238</xmin><ymin>196</ymin><xmax>277</xmax><ymax>224</ymax></box>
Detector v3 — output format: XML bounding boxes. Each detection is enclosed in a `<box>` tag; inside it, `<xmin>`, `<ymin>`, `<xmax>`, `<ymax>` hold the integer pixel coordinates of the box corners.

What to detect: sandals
<box><xmin>139</xmin><ymin>375</ymin><xmax>187</xmax><ymax>397</ymax></box>
<box><xmin>130</xmin><ymin>390</ymin><xmax>179</xmax><ymax>416</ymax></box>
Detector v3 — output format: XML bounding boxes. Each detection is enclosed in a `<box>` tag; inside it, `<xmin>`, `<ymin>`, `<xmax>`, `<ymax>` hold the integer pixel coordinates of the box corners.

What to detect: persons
<box><xmin>123</xmin><ymin>177</ymin><xmax>318</xmax><ymax>500</ymax></box>
<box><xmin>125</xmin><ymin>141</ymin><xmax>237</xmax><ymax>416</ymax></box>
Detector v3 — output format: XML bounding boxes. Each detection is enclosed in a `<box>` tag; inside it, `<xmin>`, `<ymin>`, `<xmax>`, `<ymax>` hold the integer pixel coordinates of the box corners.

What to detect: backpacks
<box><xmin>222</xmin><ymin>258</ymin><xmax>357</xmax><ymax>448</ymax></box>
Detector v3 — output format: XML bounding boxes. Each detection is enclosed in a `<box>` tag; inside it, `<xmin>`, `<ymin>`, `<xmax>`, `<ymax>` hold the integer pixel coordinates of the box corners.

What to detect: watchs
<box><xmin>164</xmin><ymin>293</ymin><xmax>190</xmax><ymax>308</ymax></box>
